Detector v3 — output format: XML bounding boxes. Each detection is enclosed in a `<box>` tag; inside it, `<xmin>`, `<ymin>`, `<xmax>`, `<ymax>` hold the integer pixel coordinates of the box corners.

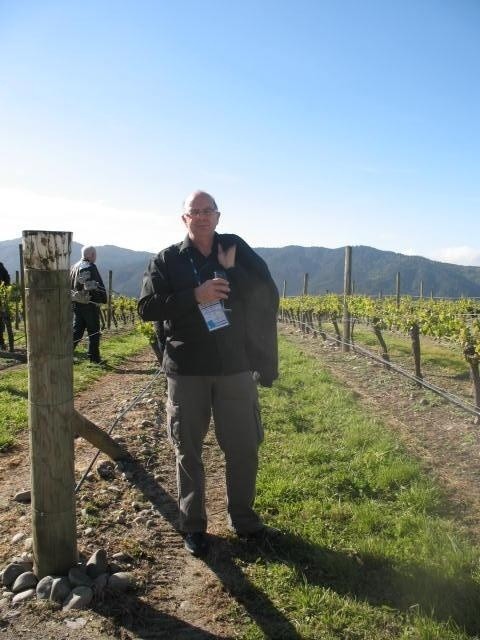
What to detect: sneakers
<box><xmin>184</xmin><ymin>533</ymin><xmax>204</xmax><ymax>555</ymax></box>
<box><xmin>236</xmin><ymin>526</ymin><xmax>281</xmax><ymax>541</ymax></box>
<box><xmin>90</xmin><ymin>360</ymin><xmax>104</xmax><ymax>364</ymax></box>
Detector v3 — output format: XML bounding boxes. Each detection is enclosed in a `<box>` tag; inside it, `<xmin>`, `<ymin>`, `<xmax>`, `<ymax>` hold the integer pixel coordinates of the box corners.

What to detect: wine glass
<box><xmin>210</xmin><ymin>271</ymin><xmax>232</xmax><ymax>312</ymax></box>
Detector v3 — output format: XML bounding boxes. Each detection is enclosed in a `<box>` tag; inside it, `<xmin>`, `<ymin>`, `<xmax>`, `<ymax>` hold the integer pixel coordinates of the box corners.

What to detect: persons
<box><xmin>72</xmin><ymin>246</ymin><xmax>108</xmax><ymax>363</ymax></box>
<box><xmin>138</xmin><ymin>191</ymin><xmax>279</xmax><ymax>555</ymax></box>
<box><xmin>0</xmin><ymin>263</ymin><xmax>11</xmax><ymax>354</ymax></box>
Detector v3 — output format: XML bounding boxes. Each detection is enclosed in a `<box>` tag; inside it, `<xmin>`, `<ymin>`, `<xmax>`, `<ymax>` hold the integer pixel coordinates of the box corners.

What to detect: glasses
<box><xmin>185</xmin><ymin>208</ymin><xmax>216</xmax><ymax>219</ymax></box>
